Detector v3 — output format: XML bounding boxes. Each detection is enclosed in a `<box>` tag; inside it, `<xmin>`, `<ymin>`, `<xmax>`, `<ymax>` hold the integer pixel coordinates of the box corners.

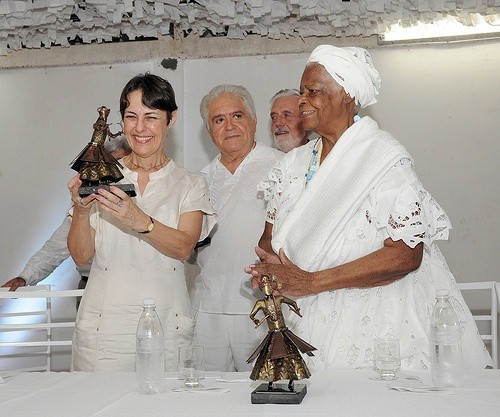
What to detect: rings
<box><xmin>117</xmin><ymin>200</ymin><xmax>123</xmax><ymax>206</ymax></box>
<box><xmin>80</xmin><ymin>199</ymin><xmax>86</xmax><ymax>206</ymax></box>
<box><xmin>272</xmin><ymin>275</ymin><xmax>277</xmax><ymax>281</ymax></box>
<box><xmin>275</xmin><ymin>283</ymin><xmax>282</xmax><ymax>290</ymax></box>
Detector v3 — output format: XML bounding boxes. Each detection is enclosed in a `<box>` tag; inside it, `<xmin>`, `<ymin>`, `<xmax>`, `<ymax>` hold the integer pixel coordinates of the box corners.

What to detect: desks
<box><xmin>0</xmin><ymin>370</ymin><xmax>500</xmax><ymax>417</ymax></box>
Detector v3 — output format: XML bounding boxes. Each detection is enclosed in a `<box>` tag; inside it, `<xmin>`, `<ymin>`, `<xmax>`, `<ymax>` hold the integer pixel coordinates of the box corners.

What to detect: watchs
<box><xmin>137</xmin><ymin>216</ymin><xmax>155</xmax><ymax>234</ymax></box>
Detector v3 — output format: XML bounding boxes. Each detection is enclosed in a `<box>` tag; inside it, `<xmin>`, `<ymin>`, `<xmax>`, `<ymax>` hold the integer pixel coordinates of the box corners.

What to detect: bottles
<box><xmin>136</xmin><ymin>300</ymin><xmax>165</xmax><ymax>396</ymax></box>
<box><xmin>429</xmin><ymin>289</ymin><xmax>463</xmax><ymax>390</ymax></box>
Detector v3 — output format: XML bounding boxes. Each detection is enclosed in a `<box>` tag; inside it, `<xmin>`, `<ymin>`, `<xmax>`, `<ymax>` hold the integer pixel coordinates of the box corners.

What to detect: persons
<box><xmin>245</xmin><ymin>45</ymin><xmax>493</xmax><ymax>372</ymax></box>
<box><xmin>71</xmin><ymin>106</ymin><xmax>123</xmax><ymax>186</ymax></box>
<box><xmin>67</xmin><ymin>71</ymin><xmax>217</xmax><ymax>371</ymax></box>
<box><xmin>246</xmin><ymin>273</ymin><xmax>318</xmax><ymax>392</ymax></box>
<box><xmin>1</xmin><ymin>136</ymin><xmax>132</xmax><ymax>312</ymax></box>
<box><xmin>268</xmin><ymin>89</ymin><xmax>313</xmax><ymax>154</ymax></box>
<box><xmin>189</xmin><ymin>85</ymin><xmax>287</xmax><ymax>373</ymax></box>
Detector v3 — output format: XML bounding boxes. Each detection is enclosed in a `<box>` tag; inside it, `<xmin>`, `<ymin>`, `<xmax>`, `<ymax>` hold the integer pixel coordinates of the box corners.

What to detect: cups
<box><xmin>373</xmin><ymin>339</ymin><xmax>400</xmax><ymax>376</ymax></box>
<box><xmin>178</xmin><ymin>346</ymin><xmax>207</xmax><ymax>389</ymax></box>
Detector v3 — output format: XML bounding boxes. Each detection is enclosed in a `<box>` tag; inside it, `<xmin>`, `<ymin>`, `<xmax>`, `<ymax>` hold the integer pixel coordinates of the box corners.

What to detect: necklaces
<box><xmin>305</xmin><ymin>115</ymin><xmax>361</xmax><ymax>182</ymax></box>
<box><xmin>129</xmin><ymin>155</ymin><xmax>166</xmax><ymax>171</ymax></box>
